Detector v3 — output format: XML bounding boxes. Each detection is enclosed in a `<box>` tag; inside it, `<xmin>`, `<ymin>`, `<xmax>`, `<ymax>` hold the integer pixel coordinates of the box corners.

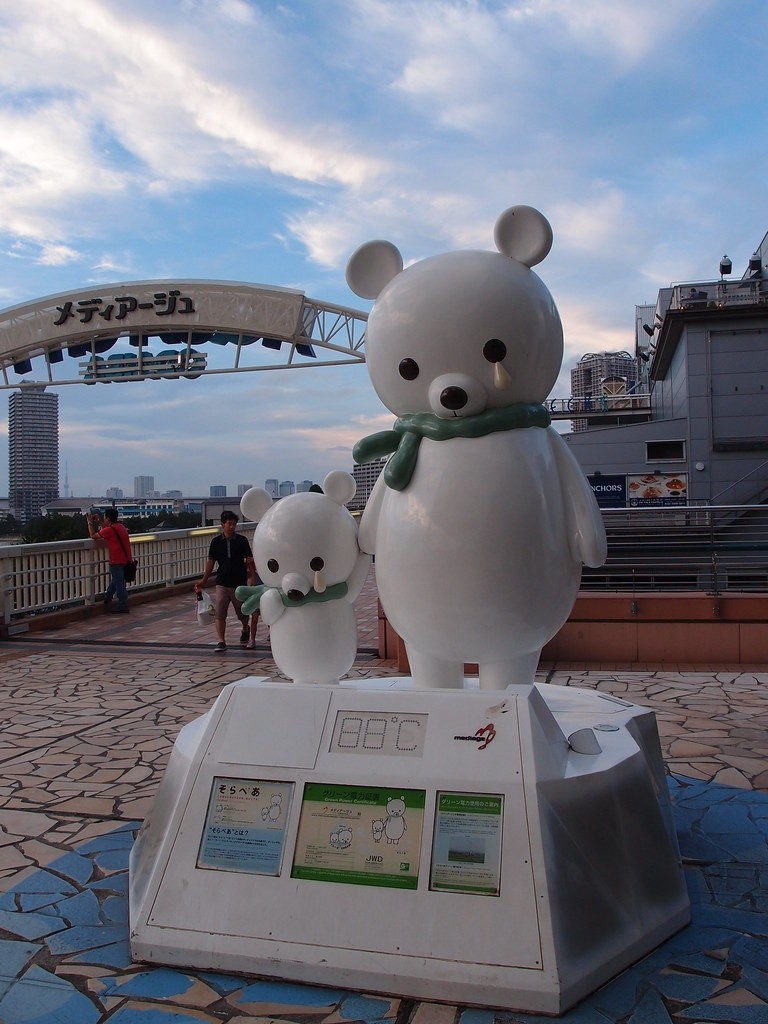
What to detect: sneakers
<box><xmin>240</xmin><ymin>626</ymin><xmax>250</xmax><ymax>642</ymax></box>
<box><xmin>215</xmin><ymin>640</ymin><xmax>227</xmax><ymax>651</ymax></box>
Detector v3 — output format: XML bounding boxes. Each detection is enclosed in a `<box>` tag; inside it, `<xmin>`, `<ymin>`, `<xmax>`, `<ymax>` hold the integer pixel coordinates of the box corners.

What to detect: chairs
<box><xmin>691</xmin><ymin>292</ymin><xmax>707</xmax><ymax>310</ymax></box>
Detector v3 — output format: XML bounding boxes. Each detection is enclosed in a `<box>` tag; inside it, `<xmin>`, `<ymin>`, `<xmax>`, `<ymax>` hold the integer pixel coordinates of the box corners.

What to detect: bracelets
<box><xmin>88</xmin><ymin>522</ymin><xmax>92</xmax><ymax>524</ymax></box>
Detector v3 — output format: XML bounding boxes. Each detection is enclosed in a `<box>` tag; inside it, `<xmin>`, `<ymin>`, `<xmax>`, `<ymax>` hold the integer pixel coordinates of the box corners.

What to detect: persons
<box><xmin>87</xmin><ymin>509</ymin><xmax>132</xmax><ymax>612</ymax></box>
<box><xmin>195</xmin><ymin>511</ymin><xmax>270</xmax><ymax>650</ymax></box>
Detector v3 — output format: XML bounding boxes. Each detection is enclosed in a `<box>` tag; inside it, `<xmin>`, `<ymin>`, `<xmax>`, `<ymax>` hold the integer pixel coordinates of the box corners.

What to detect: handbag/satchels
<box><xmin>124</xmin><ymin>561</ymin><xmax>136</xmax><ymax>582</ymax></box>
<box><xmin>196</xmin><ymin>589</ymin><xmax>215</xmax><ymax>626</ymax></box>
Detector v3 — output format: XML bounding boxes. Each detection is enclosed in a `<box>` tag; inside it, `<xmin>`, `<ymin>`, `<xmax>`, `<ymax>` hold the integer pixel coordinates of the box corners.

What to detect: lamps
<box><xmin>639</xmin><ymin>351</ymin><xmax>654</xmax><ymax>362</ymax></box>
<box><xmin>642</xmin><ymin>321</ymin><xmax>662</xmax><ymax>336</ymax></box>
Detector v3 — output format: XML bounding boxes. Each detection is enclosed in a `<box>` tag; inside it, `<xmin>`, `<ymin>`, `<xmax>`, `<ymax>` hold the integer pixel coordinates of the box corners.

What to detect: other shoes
<box><xmin>104</xmin><ymin>596</ymin><xmax>112</xmax><ymax>604</ymax></box>
<box><xmin>111</xmin><ymin>607</ymin><xmax>129</xmax><ymax>613</ymax></box>
<box><xmin>247</xmin><ymin>641</ymin><xmax>256</xmax><ymax>648</ymax></box>
